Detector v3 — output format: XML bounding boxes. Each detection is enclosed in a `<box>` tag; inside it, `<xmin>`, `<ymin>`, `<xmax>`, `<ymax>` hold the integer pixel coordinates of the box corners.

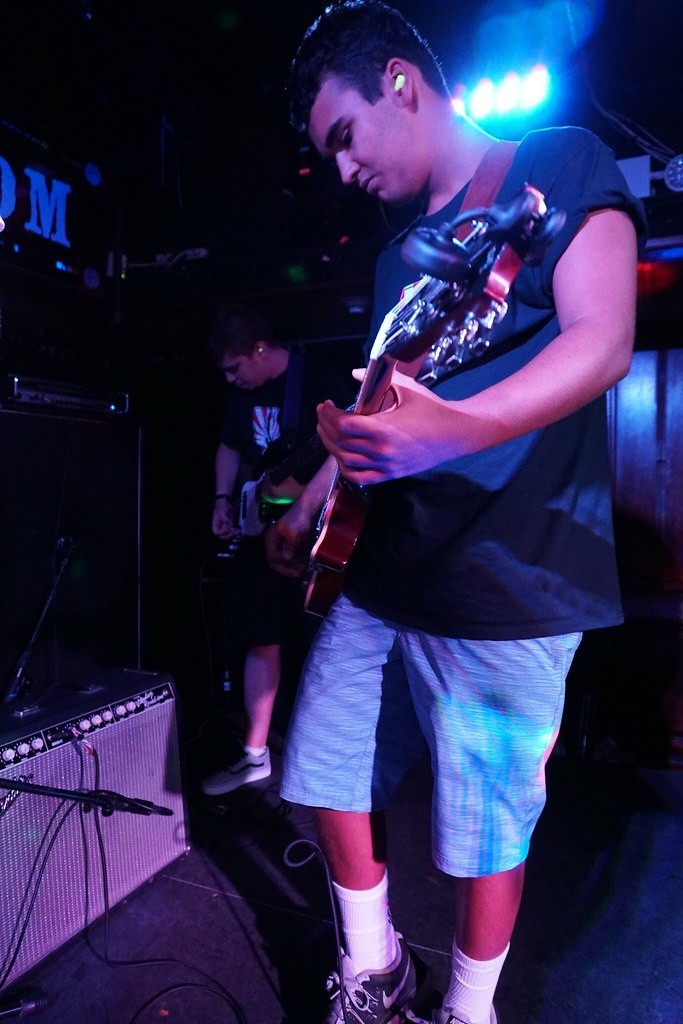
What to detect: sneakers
<box><xmin>406</xmin><ymin>996</ymin><xmax>499</xmax><ymax>1024</ymax></box>
<box><xmin>324</xmin><ymin>931</ymin><xmax>417</xmax><ymax>1024</ymax></box>
<box><xmin>200</xmin><ymin>746</ymin><xmax>272</xmax><ymax>796</ymax></box>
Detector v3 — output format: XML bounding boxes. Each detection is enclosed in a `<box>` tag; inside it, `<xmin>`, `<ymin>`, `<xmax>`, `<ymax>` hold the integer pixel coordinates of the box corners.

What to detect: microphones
<box><xmin>89</xmin><ymin>794</ymin><xmax>174</xmax><ymax>817</ymax></box>
<box><xmin>59</xmin><ymin>535</ymin><xmax>81</xmax><ymax>551</ymax></box>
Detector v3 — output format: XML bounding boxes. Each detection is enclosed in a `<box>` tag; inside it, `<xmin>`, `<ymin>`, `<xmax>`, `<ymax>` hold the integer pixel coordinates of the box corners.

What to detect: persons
<box><xmin>199</xmin><ymin>317</ymin><xmax>367</xmax><ymax>795</ymax></box>
<box><xmin>264</xmin><ymin>0</ymin><xmax>649</xmax><ymax>1024</ymax></box>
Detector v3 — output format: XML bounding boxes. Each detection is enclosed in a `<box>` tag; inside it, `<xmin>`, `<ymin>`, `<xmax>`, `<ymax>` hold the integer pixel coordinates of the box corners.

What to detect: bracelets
<box><xmin>214</xmin><ymin>493</ymin><xmax>232</xmax><ymax>501</ymax></box>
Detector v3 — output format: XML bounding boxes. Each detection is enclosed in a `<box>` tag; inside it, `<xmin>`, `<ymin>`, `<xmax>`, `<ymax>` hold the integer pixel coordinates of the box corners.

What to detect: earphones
<box><xmin>394</xmin><ymin>74</ymin><xmax>406</xmax><ymax>92</ymax></box>
<box><xmin>259</xmin><ymin>348</ymin><xmax>265</xmax><ymax>354</ymax></box>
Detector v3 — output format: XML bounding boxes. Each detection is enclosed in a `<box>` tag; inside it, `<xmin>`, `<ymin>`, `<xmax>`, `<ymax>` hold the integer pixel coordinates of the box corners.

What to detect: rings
<box><xmin>270</xmin><ymin>528</ymin><xmax>282</xmax><ymax>541</ymax></box>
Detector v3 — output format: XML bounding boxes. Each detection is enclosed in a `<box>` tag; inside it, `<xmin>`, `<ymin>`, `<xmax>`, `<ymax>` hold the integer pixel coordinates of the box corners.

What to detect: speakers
<box><xmin>0</xmin><ymin>668</ymin><xmax>190</xmax><ymax>1003</ymax></box>
<box><xmin>0</xmin><ymin>400</ymin><xmax>145</xmax><ymax>703</ymax></box>
